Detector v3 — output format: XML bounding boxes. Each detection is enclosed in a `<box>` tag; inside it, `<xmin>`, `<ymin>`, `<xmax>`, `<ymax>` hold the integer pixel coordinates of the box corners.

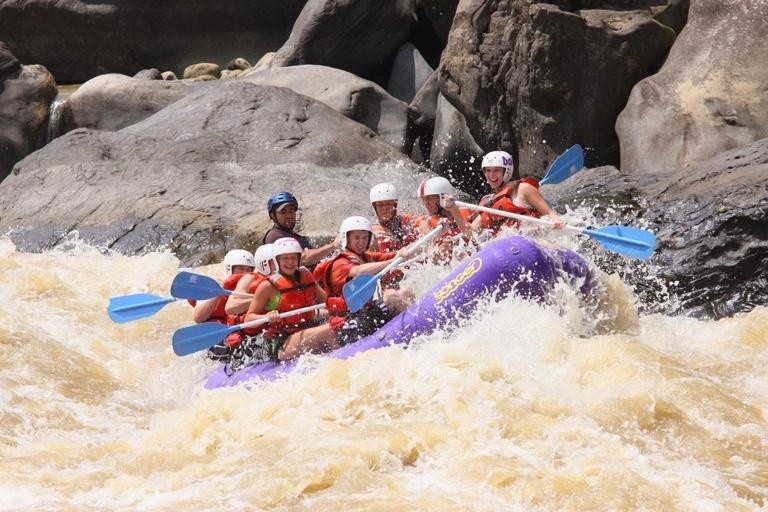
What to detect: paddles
<box><xmin>343</xmin><ymin>225</ymin><xmax>445</xmax><ymax>313</ymax></box>
<box><xmin>453</xmin><ymin>201</ymin><xmax>655</xmax><ymax>260</ymax></box>
<box><xmin>453</xmin><ymin>144</ymin><xmax>584</xmax><ymax>242</ymax></box>
<box><xmin>108</xmin><ymin>293</ymin><xmax>178</xmax><ymax>324</ymax></box>
<box><xmin>172</xmin><ymin>303</ymin><xmax>328</xmax><ymax>356</ymax></box>
<box><xmin>171</xmin><ymin>272</ymin><xmax>254</xmax><ymax>299</ymax></box>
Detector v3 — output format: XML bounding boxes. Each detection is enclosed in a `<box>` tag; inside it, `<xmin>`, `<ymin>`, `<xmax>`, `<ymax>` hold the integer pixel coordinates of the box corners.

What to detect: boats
<box><xmin>201</xmin><ymin>233</ymin><xmax>599</xmax><ymax>391</ymax></box>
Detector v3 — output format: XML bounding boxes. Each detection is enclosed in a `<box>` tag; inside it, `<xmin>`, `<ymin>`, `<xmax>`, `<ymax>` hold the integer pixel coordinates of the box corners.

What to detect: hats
<box><xmin>272</xmin><ymin>202</ymin><xmax>297</xmax><ymax>213</ymax></box>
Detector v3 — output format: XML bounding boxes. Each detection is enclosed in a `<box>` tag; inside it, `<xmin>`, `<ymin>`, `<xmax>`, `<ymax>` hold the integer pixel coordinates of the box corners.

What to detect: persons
<box><xmin>185</xmin><ymin>176</ymin><xmax>482</xmax><ymax>363</ymax></box>
<box><xmin>440</xmin><ymin>148</ymin><xmax>565</xmax><ymax>252</ymax></box>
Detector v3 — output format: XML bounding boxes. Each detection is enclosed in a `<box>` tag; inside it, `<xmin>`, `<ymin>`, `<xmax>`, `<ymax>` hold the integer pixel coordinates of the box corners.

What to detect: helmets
<box><xmin>481</xmin><ymin>150</ymin><xmax>514</xmax><ymax>183</ymax></box>
<box><xmin>370</xmin><ymin>183</ymin><xmax>398</xmax><ymax>219</ymax></box>
<box><xmin>416</xmin><ymin>175</ymin><xmax>459</xmax><ymax>202</ymax></box>
<box><xmin>267</xmin><ymin>191</ymin><xmax>298</xmax><ymax>215</ymax></box>
<box><xmin>339</xmin><ymin>216</ymin><xmax>372</xmax><ymax>252</ymax></box>
<box><xmin>223</xmin><ymin>237</ymin><xmax>303</xmax><ymax>280</ymax></box>
